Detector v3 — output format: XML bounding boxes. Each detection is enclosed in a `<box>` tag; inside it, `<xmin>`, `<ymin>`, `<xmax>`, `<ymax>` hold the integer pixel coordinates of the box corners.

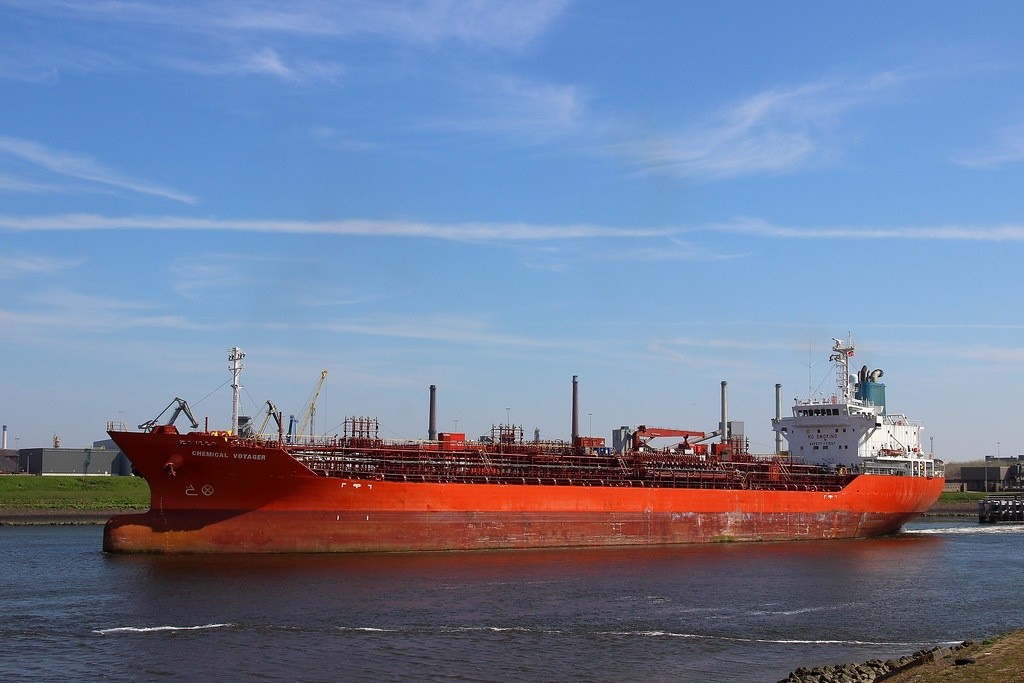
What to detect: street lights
<box><xmin>506</xmin><ymin>407</ymin><xmax>510</xmax><ymax>424</ymax></box>
<box><xmin>997</xmin><ymin>442</ymin><xmax>1000</xmax><ymax>466</ymax></box>
<box><xmin>588</xmin><ymin>414</ymin><xmax>592</xmax><ymax>438</ymax></box>
<box><xmin>985</xmin><ymin>461</ymin><xmax>991</xmax><ymax>493</ymax></box>
<box><xmin>118</xmin><ymin>410</ymin><xmax>125</xmax><ymax>432</ymax></box>
<box><xmin>15</xmin><ymin>438</ymin><xmax>19</xmax><ymax>448</ymax></box>
<box><xmin>27</xmin><ymin>453</ymin><xmax>32</xmax><ymax>474</ymax></box>
<box><xmin>454</xmin><ymin>419</ymin><xmax>458</xmax><ymax>433</ymax></box>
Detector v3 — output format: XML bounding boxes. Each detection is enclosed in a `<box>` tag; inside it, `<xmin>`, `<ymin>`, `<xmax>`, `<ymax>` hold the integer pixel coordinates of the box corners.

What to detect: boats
<box><xmin>880</xmin><ymin>449</ymin><xmax>902</xmax><ymax>455</ymax></box>
<box><xmin>101</xmin><ymin>332</ymin><xmax>945</xmax><ymax>553</ymax></box>
